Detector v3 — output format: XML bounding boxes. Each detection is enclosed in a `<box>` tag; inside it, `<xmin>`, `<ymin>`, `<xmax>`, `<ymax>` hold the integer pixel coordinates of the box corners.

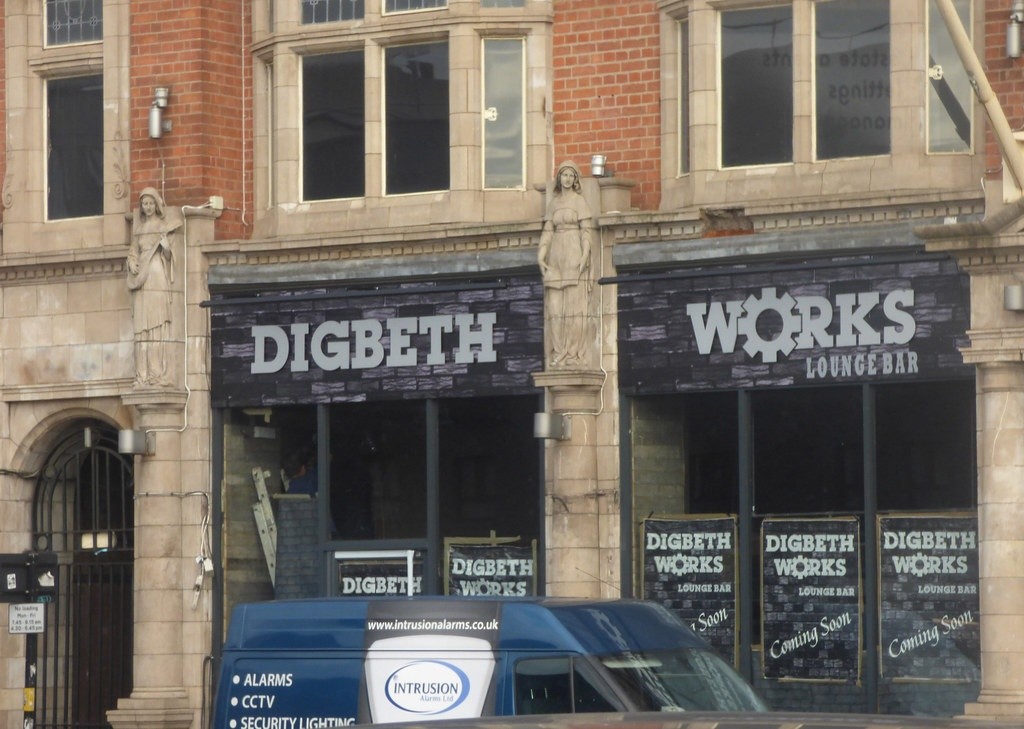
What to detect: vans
<box><xmin>209</xmin><ymin>594</ymin><xmax>765</xmax><ymax>729</ymax></box>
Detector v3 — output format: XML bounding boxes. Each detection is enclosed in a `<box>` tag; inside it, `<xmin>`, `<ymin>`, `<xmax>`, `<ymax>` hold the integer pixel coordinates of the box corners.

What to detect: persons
<box><xmin>284</xmin><ymin>454</ymin><xmax>337</xmax><ymax>532</ymax></box>
<box><xmin>122</xmin><ymin>186</ymin><xmax>178</xmax><ymax>388</ymax></box>
<box><xmin>536</xmin><ymin>160</ymin><xmax>597</xmax><ymax>372</ymax></box>
